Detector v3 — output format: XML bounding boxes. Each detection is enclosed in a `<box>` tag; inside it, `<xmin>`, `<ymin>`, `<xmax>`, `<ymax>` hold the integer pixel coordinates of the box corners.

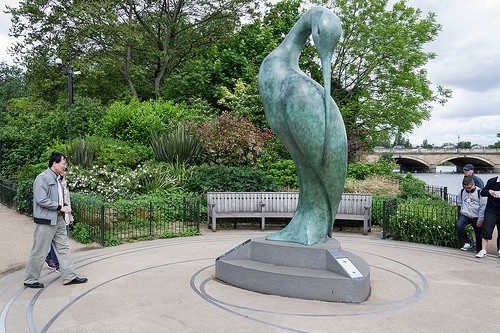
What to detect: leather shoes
<box><xmin>24</xmin><ymin>282</ymin><xmax>43</xmax><ymax>288</ymax></box>
<box><xmin>64</xmin><ymin>277</ymin><xmax>88</xmax><ymax>286</ymax></box>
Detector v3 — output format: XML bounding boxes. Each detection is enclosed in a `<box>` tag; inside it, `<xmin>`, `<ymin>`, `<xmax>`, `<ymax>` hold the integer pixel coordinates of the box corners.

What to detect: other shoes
<box><xmin>54</xmin><ymin>267</ymin><xmax>61</xmax><ymax>273</ymax></box>
<box><xmin>44</xmin><ymin>258</ymin><xmax>55</xmax><ymax>270</ymax></box>
<box><xmin>461</xmin><ymin>243</ymin><xmax>472</xmax><ymax>251</ymax></box>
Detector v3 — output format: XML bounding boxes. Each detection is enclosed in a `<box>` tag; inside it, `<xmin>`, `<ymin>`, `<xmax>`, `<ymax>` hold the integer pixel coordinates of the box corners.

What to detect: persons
<box><xmin>457</xmin><ymin>164</ymin><xmax>500</xmax><ymax>259</ymax></box>
<box><xmin>24</xmin><ymin>152</ymin><xmax>88</xmax><ymax>288</ymax></box>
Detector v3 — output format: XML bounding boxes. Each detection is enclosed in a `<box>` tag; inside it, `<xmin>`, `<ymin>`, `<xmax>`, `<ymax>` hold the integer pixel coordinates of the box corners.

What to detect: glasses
<box><xmin>463</xmin><ymin>184</ymin><xmax>474</xmax><ymax>189</ymax></box>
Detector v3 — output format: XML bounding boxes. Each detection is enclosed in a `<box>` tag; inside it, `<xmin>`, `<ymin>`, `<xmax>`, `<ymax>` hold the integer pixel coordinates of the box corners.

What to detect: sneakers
<box><xmin>476</xmin><ymin>250</ymin><xmax>487</xmax><ymax>258</ymax></box>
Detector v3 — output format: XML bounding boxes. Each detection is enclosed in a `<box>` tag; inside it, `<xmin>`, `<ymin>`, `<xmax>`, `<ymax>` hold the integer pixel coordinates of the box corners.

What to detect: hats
<box><xmin>464</xmin><ymin>164</ymin><xmax>474</xmax><ymax>171</ymax></box>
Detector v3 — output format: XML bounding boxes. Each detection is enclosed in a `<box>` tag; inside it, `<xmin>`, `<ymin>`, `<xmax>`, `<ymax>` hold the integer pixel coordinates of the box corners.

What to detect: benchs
<box><xmin>207</xmin><ymin>192</ymin><xmax>372</xmax><ymax>235</ymax></box>
